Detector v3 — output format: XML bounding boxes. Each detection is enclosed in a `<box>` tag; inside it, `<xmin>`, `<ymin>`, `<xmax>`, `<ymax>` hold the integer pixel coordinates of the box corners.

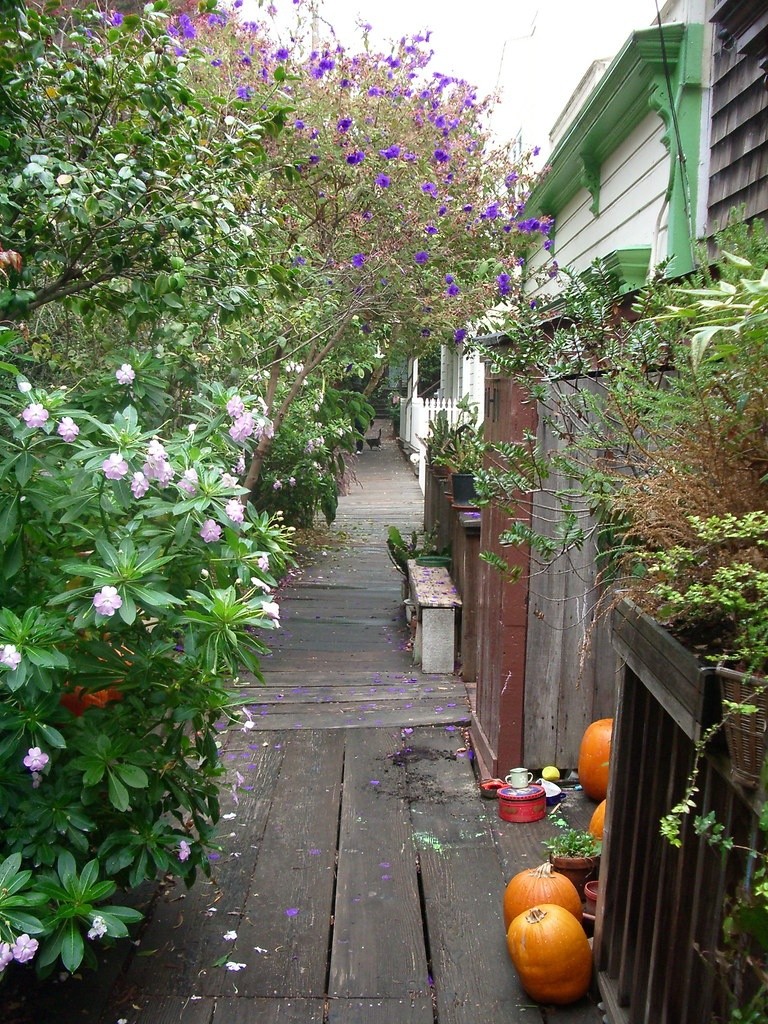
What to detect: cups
<box><xmin>504</xmin><ymin>767</ymin><xmax>533</xmax><ymax>789</ymax></box>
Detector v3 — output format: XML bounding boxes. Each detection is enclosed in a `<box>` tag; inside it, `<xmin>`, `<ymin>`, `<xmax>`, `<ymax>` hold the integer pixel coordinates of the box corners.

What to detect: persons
<box><xmin>352</xmin><ymin>415</ymin><xmax>367</xmax><ymax>455</ymax></box>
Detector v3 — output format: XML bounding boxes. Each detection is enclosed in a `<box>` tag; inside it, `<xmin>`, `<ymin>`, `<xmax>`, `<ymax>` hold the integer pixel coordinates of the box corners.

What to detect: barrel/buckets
<box><xmin>584</xmin><ymin>879</ymin><xmax>598</xmax><ymax>915</ymax></box>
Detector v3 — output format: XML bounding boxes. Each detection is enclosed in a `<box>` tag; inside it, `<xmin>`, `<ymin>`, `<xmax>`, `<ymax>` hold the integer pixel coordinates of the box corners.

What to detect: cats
<box><xmin>366</xmin><ymin>428</ymin><xmax>382</xmax><ymax>451</ymax></box>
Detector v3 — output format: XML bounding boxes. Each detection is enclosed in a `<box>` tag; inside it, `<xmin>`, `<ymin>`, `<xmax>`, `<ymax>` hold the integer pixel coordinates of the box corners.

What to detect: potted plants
<box><xmin>415</xmin><ymin>418</ymin><xmax>481</xmax><ymax>512</ymax></box>
<box><xmin>446</xmin><ymin>203</ymin><xmax>768</xmax><ymax>1024</ymax></box>
<box><xmin>386</xmin><ymin>520</ymin><xmax>453</xmax><ymax>601</ymax></box>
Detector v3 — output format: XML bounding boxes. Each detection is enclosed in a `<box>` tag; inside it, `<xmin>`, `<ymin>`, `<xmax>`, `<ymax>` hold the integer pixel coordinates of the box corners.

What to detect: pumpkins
<box><xmin>503</xmin><ymin>860</ymin><xmax>582</xmax><ymax>928</ymax></box>
<box><xmin>588</xmin><ymin>798</ymin><xmax>605</xmax><ymax>844</ymax></box>
<box><xmin>577</xmin><ymin>718</ymin><xmax>613</xmax><ymax>802</ymax></box>
<box><xmin>506</xmin><ymin>903</ymin><xmax>594</xmax><ymax>1006</ymax></box>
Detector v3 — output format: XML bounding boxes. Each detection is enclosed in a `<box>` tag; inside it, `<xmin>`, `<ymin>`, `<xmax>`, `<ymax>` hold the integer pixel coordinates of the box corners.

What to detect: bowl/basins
<box><xmin>481</xmin><ymin>779</ymin><xmax>505</xmax><ymax>798</ymax></box>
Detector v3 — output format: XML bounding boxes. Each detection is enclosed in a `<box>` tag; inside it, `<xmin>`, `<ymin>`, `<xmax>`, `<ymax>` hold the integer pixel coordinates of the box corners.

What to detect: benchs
<box><xmin>407</xmin><ymin>559</ymin><xmax>463</xmax><ymax>673</ymax></box>
